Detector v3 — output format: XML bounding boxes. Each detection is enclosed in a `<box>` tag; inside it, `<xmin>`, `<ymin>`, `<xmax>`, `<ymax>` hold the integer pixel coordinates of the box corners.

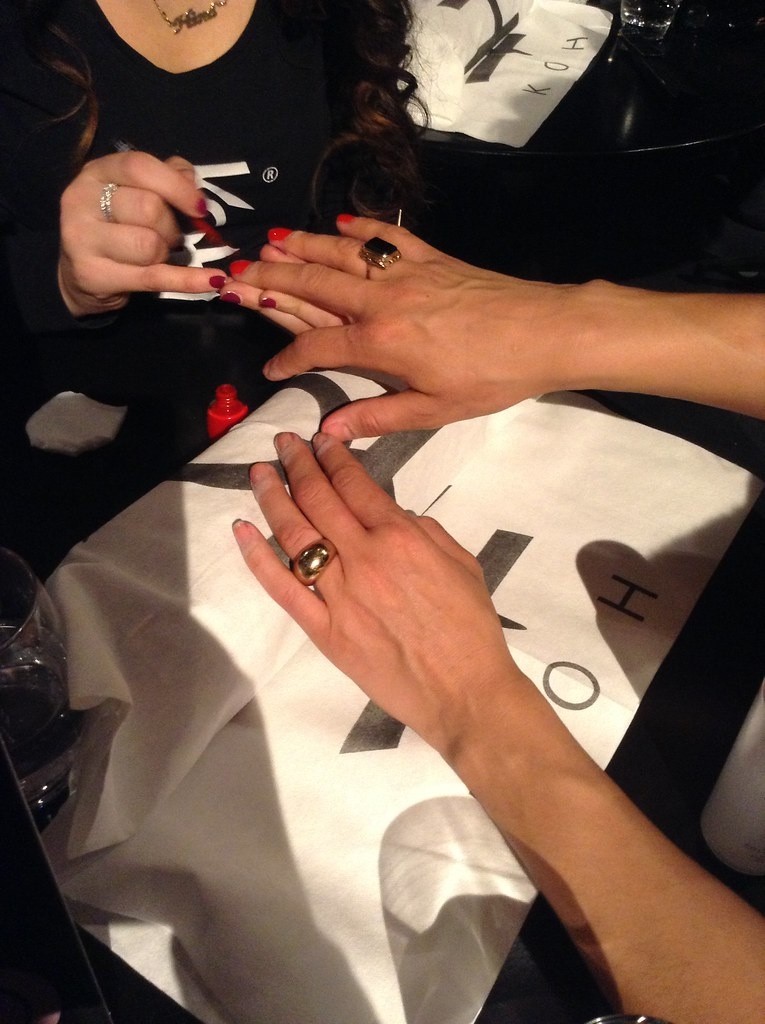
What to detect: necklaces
<box><xmin>153</xmin><ymin>0</ymin><xmax>228</xmax><ymax>34</ymax></box>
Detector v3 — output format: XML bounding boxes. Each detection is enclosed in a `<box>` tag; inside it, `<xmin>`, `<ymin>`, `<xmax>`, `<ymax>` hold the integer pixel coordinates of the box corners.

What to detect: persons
<box><xmin>0</xmin><ymin>0</ymin><xmax>765</xmax><ymax>1024</ymax></box>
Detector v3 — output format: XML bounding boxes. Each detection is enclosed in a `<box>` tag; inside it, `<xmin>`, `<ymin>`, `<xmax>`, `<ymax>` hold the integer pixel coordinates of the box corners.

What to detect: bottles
<box><xmin>206</xmin><ymin>383</ymin><xmax>247</xmax><ymax>440</ymax></box>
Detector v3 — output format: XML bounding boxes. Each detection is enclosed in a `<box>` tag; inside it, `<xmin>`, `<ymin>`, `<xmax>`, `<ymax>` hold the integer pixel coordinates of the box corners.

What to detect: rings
<box><xmin>358</xmin><ymin>236</ymin><xmax>401</xmax><ymax>279</ymax></box>
<box><xmin>290</xmin><ymin>537</ymin><xmax>337</xmax><ymax>587</ymax></box>
<box><xmin>99</xmin><ymin>180</ymin><xmax>121</xmax><ymax>223</ymax></box>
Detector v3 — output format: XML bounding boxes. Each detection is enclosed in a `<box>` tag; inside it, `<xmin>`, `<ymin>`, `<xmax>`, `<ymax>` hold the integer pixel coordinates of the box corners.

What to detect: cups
<box><xmin>0</xmin><ymin>545</ymin><xmax>71</xmax><ymax>832</ymax></box>
<box><xmin>619</xmin><ymin>0</ymin><xmax>680</xmax><ymax>57</ymax></box>
